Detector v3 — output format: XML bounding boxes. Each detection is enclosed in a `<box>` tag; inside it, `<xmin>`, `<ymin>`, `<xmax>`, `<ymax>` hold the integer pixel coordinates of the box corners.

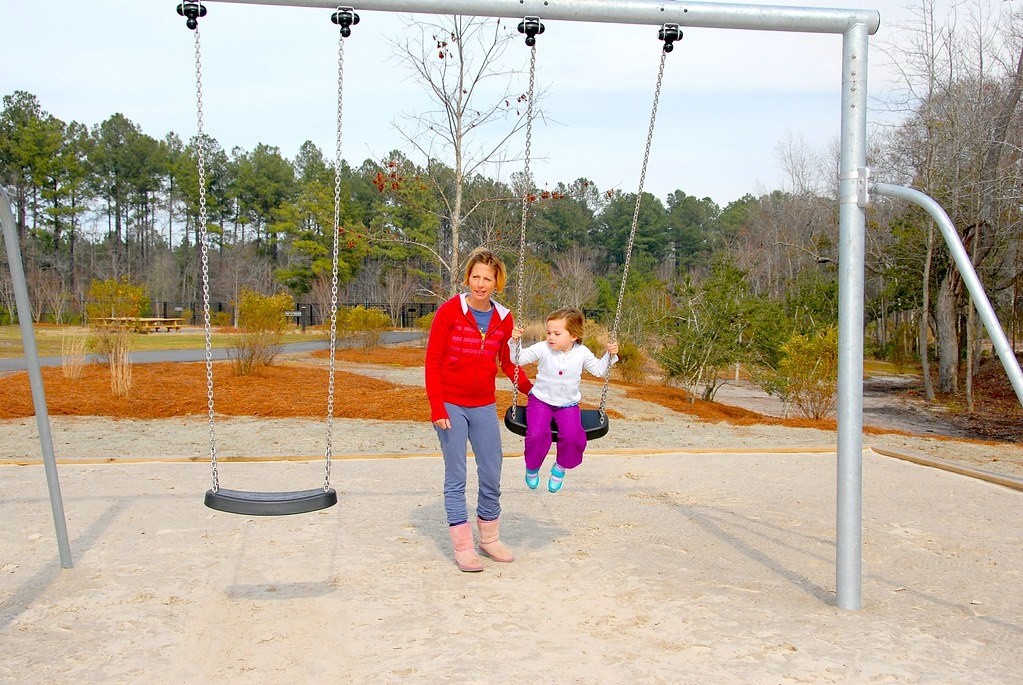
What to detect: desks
<box><xmin>91</xmin><ymin>317</ymin><xmax>185</xmax><ymax>335</ymax></box>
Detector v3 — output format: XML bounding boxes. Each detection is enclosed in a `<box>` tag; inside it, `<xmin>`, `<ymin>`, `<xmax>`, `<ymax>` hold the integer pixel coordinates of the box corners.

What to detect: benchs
<box><xmin>89</xmin><ymin>324</ymin><xmax>190</xmax><ymax>335</ymax></box>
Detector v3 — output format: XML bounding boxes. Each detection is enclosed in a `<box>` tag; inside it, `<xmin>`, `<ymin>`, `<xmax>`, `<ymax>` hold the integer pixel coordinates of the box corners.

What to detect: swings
<box><xmin>187</xmin><ymin>7</ymin><xmax>359</xmax><ymax>518</ymax></box>
<box><xmin>503</xmin><ymin>14</ymin><xmax>683</xmax><ymax>441</ymax></box>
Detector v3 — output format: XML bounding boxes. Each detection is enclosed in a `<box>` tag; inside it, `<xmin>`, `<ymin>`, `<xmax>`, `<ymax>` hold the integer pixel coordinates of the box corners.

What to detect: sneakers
<box><xmin>525</xmin><ymin>466</ymin><xmax>540</xmax><ymax>490</ymax></box>
<box><xmin>547</xmin><ymin>462</ymin><xmax>566</xmax><ymax>494</ymax></box>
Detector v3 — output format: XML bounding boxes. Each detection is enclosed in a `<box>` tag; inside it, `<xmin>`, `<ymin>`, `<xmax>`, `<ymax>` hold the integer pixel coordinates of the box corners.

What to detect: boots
<box><xmin>475</xmin><ymin>515</ymin><xmax>515</xmax><ymax>563</ymax></box>
<box><xmin>448</xmin><ymin>521</ymin><xmax>484</xmax><ymax>572</ymax></box>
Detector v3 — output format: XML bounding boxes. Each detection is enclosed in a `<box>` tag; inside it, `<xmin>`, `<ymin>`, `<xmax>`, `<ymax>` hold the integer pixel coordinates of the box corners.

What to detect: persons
<box><xmin>508</xmin><ymin>304</ymin><xmax>618</xmax><ymax>493</ymax></box>
<box><xmin>424</xmin><ymin>247</ymin><xmax>533</xmax><ymax>572</ymax></box>
